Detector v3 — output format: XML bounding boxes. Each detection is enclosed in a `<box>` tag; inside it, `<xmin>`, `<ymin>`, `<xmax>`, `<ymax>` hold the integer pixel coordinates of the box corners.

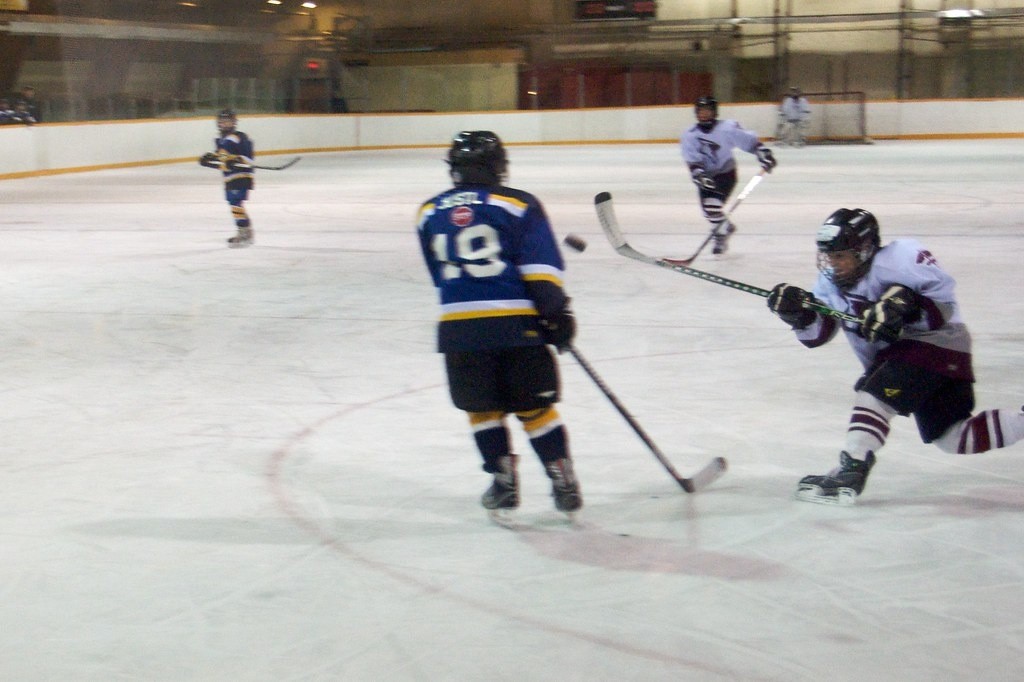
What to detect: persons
<box><xmin>768</xmin><ymin>208</ymin><xmax>1024</xmax><ymax>498</ymax></box>
<box><xmin>681</xmin><ymin>95</ymin><xmax>777</xmax><ymax>255</ymax></box>
<box><xmin>0</xmin><ymin>86</ymin><xmax>43</xmax><ymax>125</ymax></box>
<box><xmin>199</xmin><ymin>108</ymin><xmax>255</xmax><ymax>245</ymax></box>
<box><xmin>413</xmin><ymin>131</ymin><xmax>586</xmax><ymax>513</ymax></box>
<box><xmin>779</xmin><ymin>86</ymin><xmax>811</xmax><ymax>145</ymax></box>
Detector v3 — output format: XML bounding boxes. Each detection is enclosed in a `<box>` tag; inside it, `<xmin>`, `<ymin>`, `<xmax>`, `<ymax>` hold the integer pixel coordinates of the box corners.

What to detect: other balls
<box><xmin>563</xmin><ymin>233</ymin><xmax>587</xmax><ymax>253</ymax></box>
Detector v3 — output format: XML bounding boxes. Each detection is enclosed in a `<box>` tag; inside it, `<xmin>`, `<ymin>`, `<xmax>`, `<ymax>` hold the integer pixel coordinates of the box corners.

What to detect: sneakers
<box><xmin>711</xmin><ymin>239</ymin><xmax>728</xmax><ymax>254</ymax></box>
<box><xmin>479</xmin><ymin>455</ymin><xmax>521</xmax><ymax>530</ymax></box>
<box><xmin>551</xmin><ymin>481</ymin><xmax>584</xmax><ymax>522</ymax></box>
<box><xmin>227</xmin><ymin>224</ymin><xmax>255</xmax><ymax>247</ymax></box>
<box><xmin>793</xmin><ymin>449</ymin><xmax>877</xmax><ymax>507</ymax></box>
<box><xmin>713</xmin><ymin>221</ymin><xmax>736</xmax><ymax>243</ymax></box>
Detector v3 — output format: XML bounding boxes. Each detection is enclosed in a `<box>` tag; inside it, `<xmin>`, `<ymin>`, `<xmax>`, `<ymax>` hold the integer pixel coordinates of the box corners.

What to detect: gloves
<box><xmin>693</xmin><ymin>173</ymin><xmax>715</xmax><ymax>191</ymax></box>
<box><xmin>543</xmin><ymin>295</ymin><xmax>576</xmax><ymax>348</ymax></box>
<box><xmin>753</xmin><ymin>142</ymin><xmax>776</xmax><ymax>173</ymax></box>
<box><xmin>764</xmin><ymin>283</ymin><xmax>818</xmax><ymax>328</ymax></box>
<box><xmin>854</xmin><ymin>284</ymin><xmax>923</xmax><ymax>342</ymax></box>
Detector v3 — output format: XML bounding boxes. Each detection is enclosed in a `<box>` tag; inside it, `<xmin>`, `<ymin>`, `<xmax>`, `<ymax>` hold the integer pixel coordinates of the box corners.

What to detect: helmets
<box><xmin>814</xmin><ymin>207</ymin><xmax>881</xmax><ymax>289</ymax></box>
<box><xmin>693</xmin><ymin>94</ymin><xmax>718</xmax><ymax>110</ymax></box>
<box><xmin>217</xmin><ymin>109</ymin><xmax>238</xmax><ymax>122</ymax></box>
<box><xmin>442</xmin><ymin>130</ymin><xmax>509</xmax><ymax>184</ymax></box>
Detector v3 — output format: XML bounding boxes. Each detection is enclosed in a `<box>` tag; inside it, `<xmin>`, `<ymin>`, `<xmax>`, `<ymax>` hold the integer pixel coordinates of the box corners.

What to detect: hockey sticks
<box><xmin>540</xmin><ymin>318</ymin><xmax>729</xmax><ymax>495</ymax></box>
<box><xmin>593</xmin><ymin>188</ymin><xmax>906</xmax><ymax>339</ymax></box>
<box><xmin>663</xmin><ymin>164</ymin><xmax>767</xmax><ymax>266</ymax></box>
<box><xmin>206</xmin><ymin>155</ymin><xmax>302</xmax><ymax>171</ymax></box>
